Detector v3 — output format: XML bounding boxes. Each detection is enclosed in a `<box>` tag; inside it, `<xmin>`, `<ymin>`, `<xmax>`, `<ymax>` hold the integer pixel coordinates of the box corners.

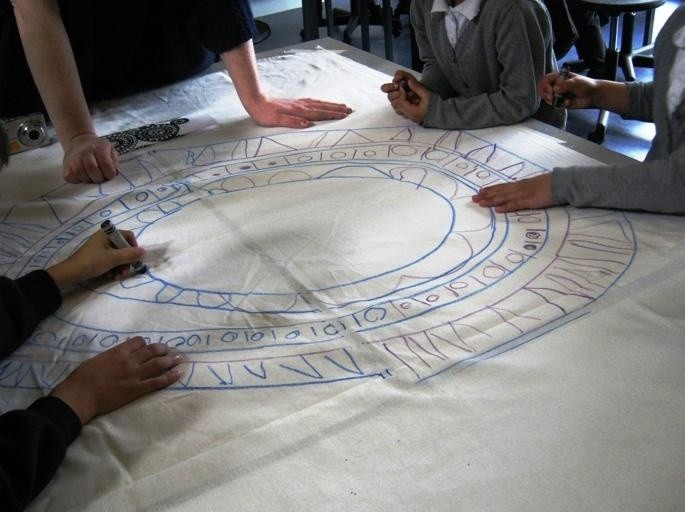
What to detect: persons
<box><xmin>471</xmin><ymin>4</ymin><xmax>685</xmax><ymax>216</ymax></box>
<box><xmin>0</xmin><ymin>227</ymin><xmax>184</xmax><ymax>512</ymax></box>
<box><xmin>380</xmin><ymin>0</ymin><xmax>567</xmax><ymax>133</ymax></box>
<box><xmin>563</xmin><ymin>1</ymin><xmax>609</xmax><ymax>80</ymax></box>
<box><xmin>3</xmin><ymin>0</ymin><xmax>353</xmax><ymax>183</ymax></box>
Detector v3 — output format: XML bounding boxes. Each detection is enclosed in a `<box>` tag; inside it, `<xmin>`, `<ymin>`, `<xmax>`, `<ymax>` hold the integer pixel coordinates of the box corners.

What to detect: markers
<box><xmin>393</xmin><ymin>78</ymin><xmax>409</xmax><ymax>91</ymax></box>
<box><xmin>552</xmin><ymin>62</ymin><xmax>572</xmax><ymax>108</ymax></box>
<box><xmin>100</xmin><ymin>219</ymin><xmax>148</xmax><ymax>274</ymax></box>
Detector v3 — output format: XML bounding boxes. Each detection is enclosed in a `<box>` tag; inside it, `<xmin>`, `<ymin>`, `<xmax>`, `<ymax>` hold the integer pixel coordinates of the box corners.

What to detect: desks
<box><xmin>0</xmin><ymin>36</ymin><xmax>685</xmax><ymax>511</ymax></box>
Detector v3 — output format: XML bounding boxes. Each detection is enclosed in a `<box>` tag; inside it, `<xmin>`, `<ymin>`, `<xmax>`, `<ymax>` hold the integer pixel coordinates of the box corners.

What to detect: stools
<box><xmin>562</xmin><ymin>0</ymin><xmax>668</xmax><ymax>143</ymax></box>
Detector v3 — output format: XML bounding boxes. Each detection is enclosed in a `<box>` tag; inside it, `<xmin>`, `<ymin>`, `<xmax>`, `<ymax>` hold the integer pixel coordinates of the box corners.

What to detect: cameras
<box><xmin>0</xmin><ymin>111</ymin><xmax>51</xmax><ymax>156</ymax></box>
